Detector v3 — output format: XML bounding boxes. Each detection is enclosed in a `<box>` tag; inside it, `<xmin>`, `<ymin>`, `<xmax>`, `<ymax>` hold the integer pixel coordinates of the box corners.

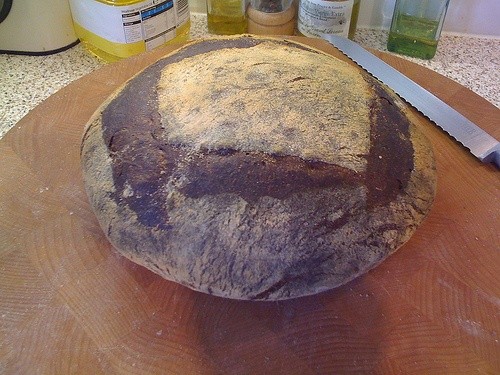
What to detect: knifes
<box><xmin>323</xmin><ymin>33</ymin><xmax>500</xmax><ymax>173</ymax></box>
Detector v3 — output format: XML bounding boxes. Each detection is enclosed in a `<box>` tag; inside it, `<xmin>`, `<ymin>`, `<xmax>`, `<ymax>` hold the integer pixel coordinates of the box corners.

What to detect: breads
<box><xmin>79</xmin><ymin>31</ymin><xmax>438</xmax><ymax>299</ymax></box>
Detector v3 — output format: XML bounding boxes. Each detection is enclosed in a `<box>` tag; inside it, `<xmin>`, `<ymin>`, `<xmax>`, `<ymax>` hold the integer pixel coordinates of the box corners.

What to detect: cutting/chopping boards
<box><xmin>0</xmin><ymin>37</ymin><xmax>500</xmax><ymax>375</ymax></box>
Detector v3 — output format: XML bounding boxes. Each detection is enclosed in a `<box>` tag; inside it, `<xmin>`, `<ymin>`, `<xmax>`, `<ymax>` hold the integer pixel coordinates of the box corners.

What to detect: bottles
<box><xmin>68</xmin><ymin>0</ymin><xmax>190</xmax><ymax>65</ymax></box>
<box><xmin>294</xmin><ymin>0</ymin><xmax>360</xmax><ymax>40</ymax></box>
<box><xmin>207</xmin><ymin>0</ymin><xmax>247</xmax><ymax>36</ymax></box>
<box><xmin>387</xmin><ymin>0</ymin><xmax>450</xmax><ymax>59</ymax></box>
